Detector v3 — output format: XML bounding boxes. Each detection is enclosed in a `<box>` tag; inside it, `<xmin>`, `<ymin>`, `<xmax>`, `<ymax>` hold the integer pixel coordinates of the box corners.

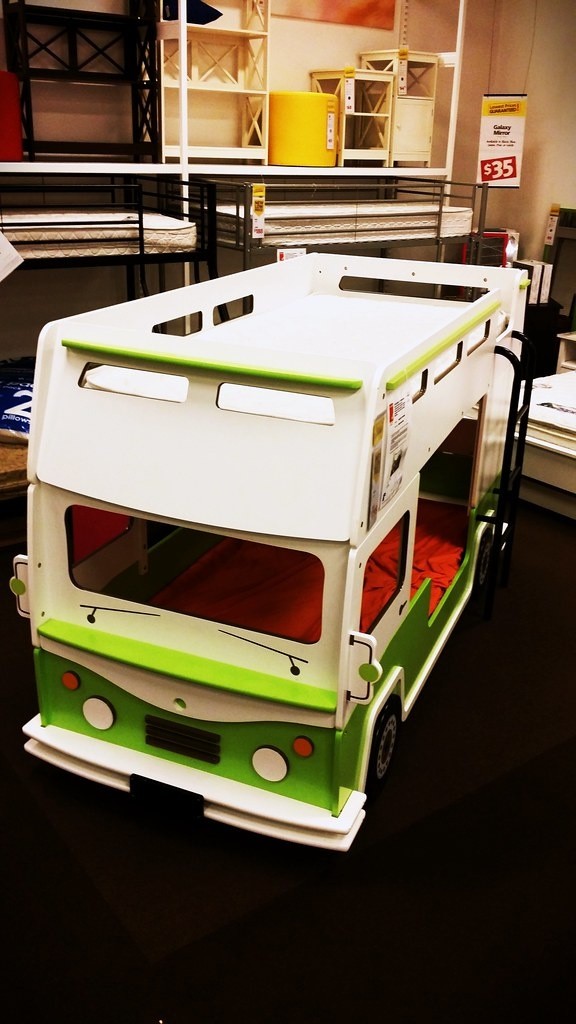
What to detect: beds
<box><xmin>512</xmin><ymin>370</ymin><xmax>576</xmax><ymax>521</ymax></box>
<box><xmin>0</xmin><ymin>179</ymin><xmax>489</xmax><ymax>549</ymax></box>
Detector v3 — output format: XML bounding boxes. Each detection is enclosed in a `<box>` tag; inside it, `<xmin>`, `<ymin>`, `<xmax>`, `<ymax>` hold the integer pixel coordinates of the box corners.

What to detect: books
<box><xmin>512</xmin><ymin>260</ymin><xmax>554</xmax><ymax>306</ymax></box>
<box><xmin>513</xmin><ymin>370</ymin><xmax>576</xmax><ymax>452</ymax></box>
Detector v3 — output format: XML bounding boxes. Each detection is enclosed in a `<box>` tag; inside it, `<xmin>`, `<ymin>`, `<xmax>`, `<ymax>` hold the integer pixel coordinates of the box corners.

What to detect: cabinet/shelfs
<box><xmin>555</xmin><ymin>331</ymin><xmax>576</xmax><ymax>374</ymax></box>
<box><xmin>0</xmin><ymin>0</ymin><xmax>438</xmax><ymax>168</ymax></box>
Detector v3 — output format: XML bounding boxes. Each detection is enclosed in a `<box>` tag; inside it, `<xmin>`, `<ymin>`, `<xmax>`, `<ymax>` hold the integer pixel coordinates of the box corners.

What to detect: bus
<box><xmin>9</xmin><ymin>252</ymin><xmax>536</xmax><ymax>851</ymax></box>
